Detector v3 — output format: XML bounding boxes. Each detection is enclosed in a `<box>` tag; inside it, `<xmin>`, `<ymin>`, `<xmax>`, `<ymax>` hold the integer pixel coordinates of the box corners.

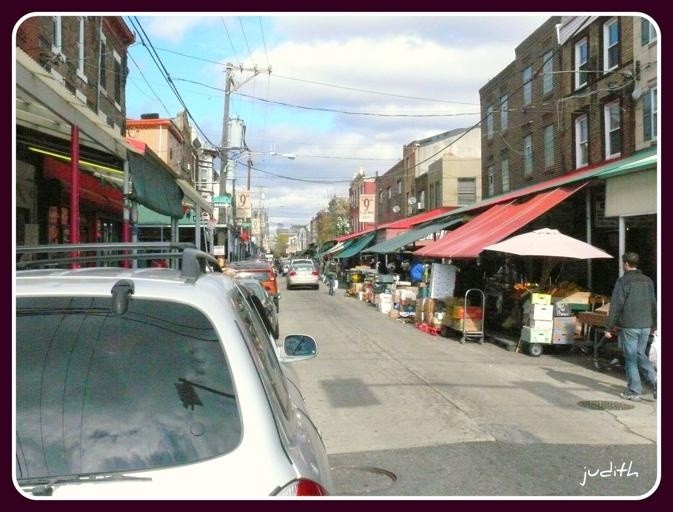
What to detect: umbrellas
<box><xmin>482</xmin><ymin>227</ymin><xmax>614</xmax><ymax>260</ymax></box>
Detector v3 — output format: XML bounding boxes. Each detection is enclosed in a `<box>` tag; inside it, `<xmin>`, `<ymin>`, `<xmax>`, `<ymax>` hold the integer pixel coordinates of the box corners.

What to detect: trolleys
<box><xmin>441</xmin><ymin>288</ymin><xmax>485</xmax><ymax>344</ymax></box>
<box><xmin>523</xmin><ymin>295</ymin><xmax>604</xmax><ymax>356</ymax></box>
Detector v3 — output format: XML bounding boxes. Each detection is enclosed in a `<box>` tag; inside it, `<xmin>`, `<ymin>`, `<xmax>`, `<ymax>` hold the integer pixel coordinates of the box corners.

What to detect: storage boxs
<box><xmin>350</xmin><ymin>272</ymin><xmax>577</xmax><ymax>345</ymax></box>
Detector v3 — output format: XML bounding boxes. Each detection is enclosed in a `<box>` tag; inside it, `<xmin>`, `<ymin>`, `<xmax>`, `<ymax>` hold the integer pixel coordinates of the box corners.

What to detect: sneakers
<box><xmin>619</xmin><ymin>389</ymin><xmax>642</xmax><ymax>402</ymax></box>
<box><xmin>652</xmin><ymin>379</ymin><xmax>657</xmax><ymax>400</ymax></box>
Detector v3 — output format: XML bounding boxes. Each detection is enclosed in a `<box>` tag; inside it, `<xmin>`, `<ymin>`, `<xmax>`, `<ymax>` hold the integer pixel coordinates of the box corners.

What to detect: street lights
<box><xmin>215</xmin><ymin>152</ymin><xmax>296</xmax><ymax>269</ymax></box>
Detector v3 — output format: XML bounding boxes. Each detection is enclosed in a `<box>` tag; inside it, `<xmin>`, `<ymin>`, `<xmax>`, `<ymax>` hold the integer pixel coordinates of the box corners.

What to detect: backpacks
<box><xmin>329</xmin><ymin>260</ymin><xmax>337</xmax><ymax>272</ymax></box>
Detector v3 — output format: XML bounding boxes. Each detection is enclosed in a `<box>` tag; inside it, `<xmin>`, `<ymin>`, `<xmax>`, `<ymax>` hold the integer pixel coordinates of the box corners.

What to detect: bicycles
<box><xmin>324</xmin><ymin>273</ymin><xmax>334</xmax><ymax>296</ymax></box>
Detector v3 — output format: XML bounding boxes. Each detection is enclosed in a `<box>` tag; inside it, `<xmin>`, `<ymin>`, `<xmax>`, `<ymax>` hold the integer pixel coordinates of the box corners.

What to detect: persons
<box><xmin>604</xmin><ymin>252</ymin><xmax>657</xmax><ymax>401</ymax></box>
<box><xmin>496</xmin><ymin>256</ymin><xmax>517</xmax><ymax>286</ymax></box>
<box><xmin>324</xmin><ymin>255</ymin><xmax>337</xmax><ymax>294</ymax></box>
